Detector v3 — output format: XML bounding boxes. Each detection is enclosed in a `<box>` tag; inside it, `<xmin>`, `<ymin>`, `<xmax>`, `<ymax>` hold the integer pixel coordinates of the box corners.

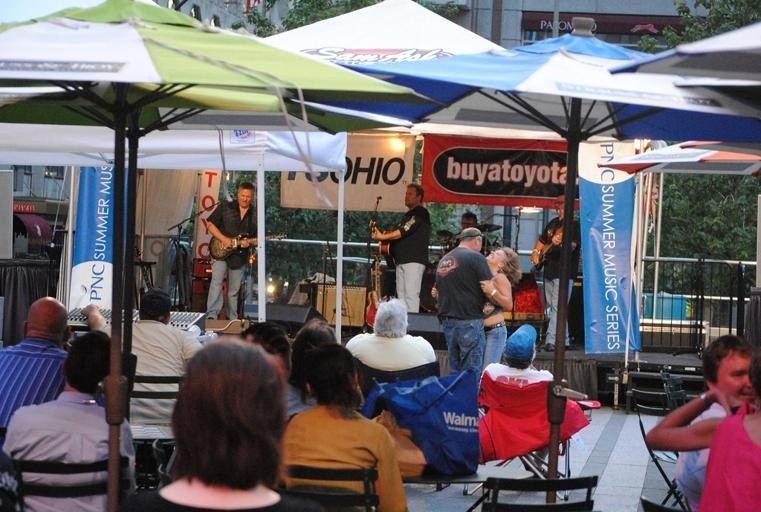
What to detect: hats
<box><xmin>505</xmin><ymin>324</ymin><xmax>537</xmax><ymax>360</ymax></box>
<box><xmin>141</xmin><ymin>287</ymin><xmax>171</xmax><ymax>319</ymax></box>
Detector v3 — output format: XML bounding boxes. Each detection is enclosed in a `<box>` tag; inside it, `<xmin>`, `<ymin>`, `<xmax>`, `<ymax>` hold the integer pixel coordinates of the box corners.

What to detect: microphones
<box><xmin>376</xmin><ymin>196</ymin><xmax>382</xmax><ymax>205</ymax></box>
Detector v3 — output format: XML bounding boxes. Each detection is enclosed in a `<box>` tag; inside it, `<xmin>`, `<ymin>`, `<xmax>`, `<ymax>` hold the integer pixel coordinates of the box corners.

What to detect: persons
<box><xmin>207</xmin><ymin>181</ymin><xmax>257</xmax><ymax>320</ymax></box>
<box><xmin>370</xmin><ymin>184</ymin><xmax>582</xmax><ymax>377</ymax></box>
<box><xmin>1</xmin><ymin>289</ymin><xmax>552</xmax><ymax>512</ymax></box>
<box><xmin>645</xmin><ymin>335</ymin><xmax>761</xmax><ymax>512</ymax></box>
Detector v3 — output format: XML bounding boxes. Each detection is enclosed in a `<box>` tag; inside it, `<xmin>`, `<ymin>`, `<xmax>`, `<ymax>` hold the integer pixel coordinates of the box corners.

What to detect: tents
<box><xmin>0</xmin><ymin>123</ymin><xmax>348</xmax><ymax>343</ymax></box>
<box><xmin>262</xmin><ymin>1</ymin><xmax>649</xmax><ymax>317</ymax></box>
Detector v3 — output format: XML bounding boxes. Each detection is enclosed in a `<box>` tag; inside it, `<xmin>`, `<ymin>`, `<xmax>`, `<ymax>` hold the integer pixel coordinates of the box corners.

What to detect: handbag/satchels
<box><xmin>371</xmin><ymin>411</ymin><xmax>428</xmax><ymax>479</ymax></box>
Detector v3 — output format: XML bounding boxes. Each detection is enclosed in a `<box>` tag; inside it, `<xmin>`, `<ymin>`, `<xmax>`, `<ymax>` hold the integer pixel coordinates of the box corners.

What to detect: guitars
<box><xmin>209</xmin><ymin>233</ymin><xmax>286</xmax><ymax>261</ymax></box>
<box><xmin>368</xmin><ymin>219</ymin><xmax>395</xmax><ymax>269</ymax></box>
<box><xmin>364</xmin><ymin>254</ymin><xmax>390</xmax><ymax>328</ymax></box>
<box><xmin>532</xmin><ymin>227</ymin><xmax>565</xmax><ymax>269</ymax></box>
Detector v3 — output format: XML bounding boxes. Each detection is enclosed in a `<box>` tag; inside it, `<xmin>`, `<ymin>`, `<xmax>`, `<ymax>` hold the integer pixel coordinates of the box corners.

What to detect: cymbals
<box><xmin>477</xmin><ymin>224</ymin><xmax>501</xmax><ymax>231</ymax></box>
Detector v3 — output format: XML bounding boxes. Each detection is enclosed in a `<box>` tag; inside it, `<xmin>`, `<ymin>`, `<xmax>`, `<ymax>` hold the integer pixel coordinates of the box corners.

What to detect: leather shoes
<box><xmin>545</xmin><ymin>343</ymin><xmax>555</xmax><ymax>352</ymax></box>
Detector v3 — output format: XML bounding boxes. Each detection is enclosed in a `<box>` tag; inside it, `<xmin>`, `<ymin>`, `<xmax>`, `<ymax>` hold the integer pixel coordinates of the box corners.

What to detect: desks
<box><xmin>403</xmin><ymin>465</ymin><xmax>532</xmax><ymax>485</ymax></box>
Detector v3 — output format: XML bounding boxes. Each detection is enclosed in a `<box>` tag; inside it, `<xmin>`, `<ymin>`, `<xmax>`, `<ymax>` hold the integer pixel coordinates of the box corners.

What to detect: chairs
<box><xmin>667</xmin><ymin>377</ymin><xmax>687</xmax><ymax>406</ymax></box>
<box><xmin>462</xmin><ymin>369</ymin><xmax>600</xmax><ymax>501</ymax></box>
<box><xmin>353</xmin><ymin>357</ymin><xmax>440</xmax><ymax>402</ymax></box>
<box><xmin>661</xmin><ymin>364</ymin><xmax>679</xmax><ymax>408</ymax></box>
<box><xmin>10</xmin><ymin>458</ymin><xmax>127</xmax><ymax>511</ymax></box>
<box><xmin>123</xmin><ymin>374</ymin><xmax>183</xmax><ymax>482</ymax></box>
<box><xmin>279</xmin><ymin>464</ymin><xmax>380</xmax><ymax>512</ymax></box>
<box><xmin>481</xmin><ymin>475</ymin><xmax>598</xmax><ymax>512</ymax></box>
<box><xmin>631</xmin><ymin>389</ymin><xmax>690</xmax><ymax>512</ymax></box>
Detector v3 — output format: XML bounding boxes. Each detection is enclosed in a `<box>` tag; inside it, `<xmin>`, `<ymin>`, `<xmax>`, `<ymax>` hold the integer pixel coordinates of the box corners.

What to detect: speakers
<box><xmin>266</xmin><ymin>302</ymin><xmax>328</xmax><ymax>331</ymax></box>
<box><xmin>407</xmin><ymin>311</ymin><xmax>447</xmax><ymax>350</ymax></box>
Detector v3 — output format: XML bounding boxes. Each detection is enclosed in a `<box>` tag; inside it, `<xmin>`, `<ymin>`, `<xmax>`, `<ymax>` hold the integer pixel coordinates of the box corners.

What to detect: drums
<box><xmin>419</xmin><ymin>261</ymin><xmax>440</xmax><ymax>312</ymax></box>
